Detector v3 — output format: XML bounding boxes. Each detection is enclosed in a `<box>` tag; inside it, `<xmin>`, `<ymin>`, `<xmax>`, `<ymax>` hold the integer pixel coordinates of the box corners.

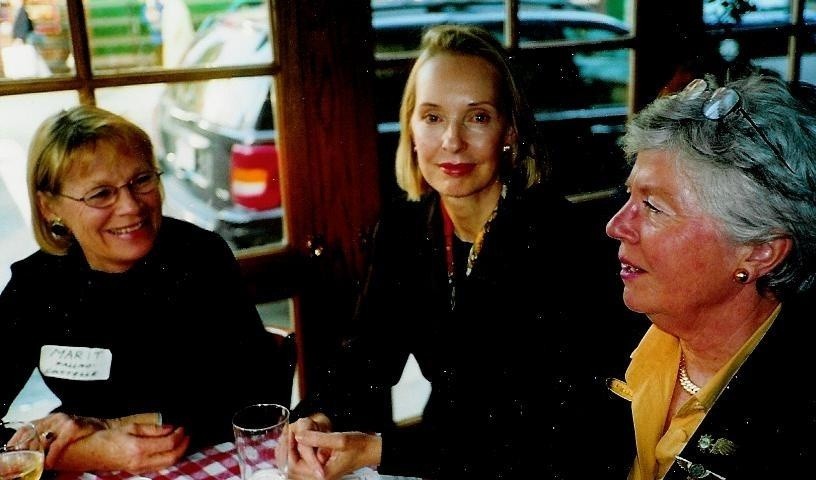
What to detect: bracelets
<box><xmin>88</xmin><ymin>416</ymin><xmax>110</xmax><ymax>433</ymax></box>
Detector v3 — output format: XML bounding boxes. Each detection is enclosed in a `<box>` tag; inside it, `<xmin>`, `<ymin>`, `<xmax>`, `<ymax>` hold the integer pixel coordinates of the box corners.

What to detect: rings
<box><xmin>40</xmin><ymin>430</ymin><xmax>55</xmax><ymax>444</ymax></box>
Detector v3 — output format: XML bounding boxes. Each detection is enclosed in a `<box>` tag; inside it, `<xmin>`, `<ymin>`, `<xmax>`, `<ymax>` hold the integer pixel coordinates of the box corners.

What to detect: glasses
<box><xmin>56</xmin><ymin>171</ymin><xmax>164</xmax><ymax>210</ymax></box>
<box><xmin>684</xmin><ymin>79</ymin><xmax>794</xmax><ymax>172</ymax></box>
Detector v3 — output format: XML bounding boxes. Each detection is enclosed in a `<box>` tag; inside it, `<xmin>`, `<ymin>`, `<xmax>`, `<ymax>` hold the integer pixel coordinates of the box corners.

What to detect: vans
<box><xmin>153</xmin><ymin>0</ymin><xmax>816</xmax><ymax>254</ymax></box>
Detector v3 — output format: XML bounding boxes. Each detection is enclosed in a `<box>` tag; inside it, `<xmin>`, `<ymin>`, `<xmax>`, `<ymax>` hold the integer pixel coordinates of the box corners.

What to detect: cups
<box><xmin>232</xmin><ymin>403</ymin><xmax>289</xmax><ymax>479</ymax></box>
<box><xmin>0</xmin><ymin>422</ymin><xmax>44</xmax><ymax>480</ymax></box>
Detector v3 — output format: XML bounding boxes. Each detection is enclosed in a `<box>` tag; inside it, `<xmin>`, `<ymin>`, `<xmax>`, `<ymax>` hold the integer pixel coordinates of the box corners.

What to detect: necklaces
<box><xmin>677</xmin><ymin>354</ymin><xmax>701</xmax><ymax>396</ymax></box>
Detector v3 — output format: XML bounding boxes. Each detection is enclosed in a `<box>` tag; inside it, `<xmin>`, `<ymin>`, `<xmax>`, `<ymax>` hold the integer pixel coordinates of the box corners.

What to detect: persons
<box><xmin>1</xmin><ymin>103</ymin><xmax>278</xmax><ymax>477</ymax></box>
<box><xmin>597</xmin><ymin>70</ymin><xmax>814</xmax><ymax>479</ymax></box>
<box><xmin>275</xmin><ymin>26</ymin><xmax>624</xmax><ymax>479</ymax></box>
